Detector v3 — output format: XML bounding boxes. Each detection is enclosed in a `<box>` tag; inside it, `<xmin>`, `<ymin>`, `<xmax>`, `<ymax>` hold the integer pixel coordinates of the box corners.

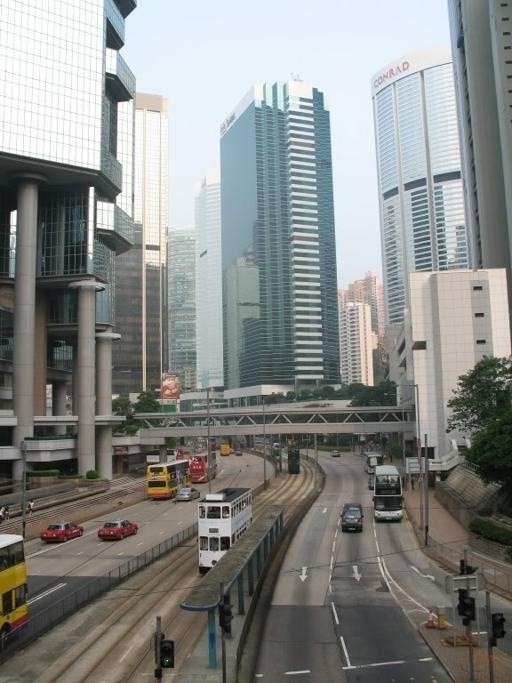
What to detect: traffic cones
<box><xmin>424</xmin><ymin>609</ymin><xmax>436</xmax><ymax>628</ymax></box>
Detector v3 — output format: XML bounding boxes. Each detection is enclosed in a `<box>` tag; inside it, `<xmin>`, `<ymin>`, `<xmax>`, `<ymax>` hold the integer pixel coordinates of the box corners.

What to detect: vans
<box><xmin>340</xmin><ymin>503</ymin><xmax>365</xmax><ymax>534</ymax></box>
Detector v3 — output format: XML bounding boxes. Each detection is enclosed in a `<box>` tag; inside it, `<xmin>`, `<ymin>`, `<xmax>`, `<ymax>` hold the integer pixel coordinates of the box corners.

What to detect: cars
<box><xmin>230</xmin><ymin>448</ymin><xmax>243</xmax><ymax>456</ymax></box>
<box><xmin>97</xmin><ymin>517</ymin><xmax>139</xmax><ymax>542</ymax></box>
<box><xmin>330</xmin><ymin>447</ymin><xmax>342</xmax><ymax>459</ymax></box>
<box><xmin>175</xmin><ymin>488</ymin><xmax>201</xmax><ymax>501</ymax></box>
<box><xmin>41</xmin><ymin>521</ymin><xmax>84</xmax><ymax>543</ymax></box>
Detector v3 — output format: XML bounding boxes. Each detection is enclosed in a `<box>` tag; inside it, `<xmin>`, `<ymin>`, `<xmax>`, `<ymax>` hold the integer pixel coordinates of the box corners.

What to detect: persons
<box><xmin>0</xmin><ymin>506</ymin><xmax>6</xmax><ymax>523</ymax></box>
<box><xmin>222</xmin><ymin>507</ymin><xmax>229</xmax><ymax>518</ymax></box>
<box><xmin>5</xmin><ymin>503</ymin><xmax>10</xmax><ymax>520</ymax></box>
<box><xmin>28</xmin><ymin>498</ymin><xmax>35</xmax><ymax>519</ymax></box>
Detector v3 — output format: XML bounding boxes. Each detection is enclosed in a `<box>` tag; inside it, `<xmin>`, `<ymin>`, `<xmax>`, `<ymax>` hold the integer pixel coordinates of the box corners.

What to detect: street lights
<box><xmin>393</xmin><ymin>382</ymin><xmax>425</xmax><ymax>530</ymax></box>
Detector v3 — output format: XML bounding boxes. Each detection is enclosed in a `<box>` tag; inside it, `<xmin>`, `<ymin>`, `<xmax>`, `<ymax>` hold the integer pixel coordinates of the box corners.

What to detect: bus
<box><xmin>189</xmin><ymin>452</ymin><xmax>217</xmax><ymax>484</ymax></box>
<box><xmin>1</xmin><ymin>533</ymin><xmax>30</xmax><ymax>643</ymax></box>
<box><xmin>364</xmin><ymin>452</ymin><xmax>385</xmax><ymax>474</ymax></box>
<box><xmin>145</xmin><ymin>459</ymin><xmax>190</xmax><ymax>502</ymax></box>
<box><xmin>198</xmin><ymin>486</ymin><xmax>255</xmax><ymax>575</ymax></box>
<box><xmin>372</xmin><ymin>465</ymin><xmax>404</xmax><ymax>523</ymax></box>
<box><xmin>220</xmin><ymin>442</ymin><xmax>230</xmax><ymax>456</ymax></box>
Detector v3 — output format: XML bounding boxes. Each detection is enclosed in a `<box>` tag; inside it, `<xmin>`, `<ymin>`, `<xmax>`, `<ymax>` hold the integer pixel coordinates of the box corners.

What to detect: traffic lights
<box><xmin>490</xmin><ymin>612</ymin><xmax>506</xmax><ymax>639</ymax></box>
<box><xmin>463</xmin><ymin>596</ymin><xmax>477</xmax><ymax>620</ymax></box>
<box><xmin>160</xmin><ymin>639</ymin><xmax>177</xmax><ymax>669</ymax></box>
